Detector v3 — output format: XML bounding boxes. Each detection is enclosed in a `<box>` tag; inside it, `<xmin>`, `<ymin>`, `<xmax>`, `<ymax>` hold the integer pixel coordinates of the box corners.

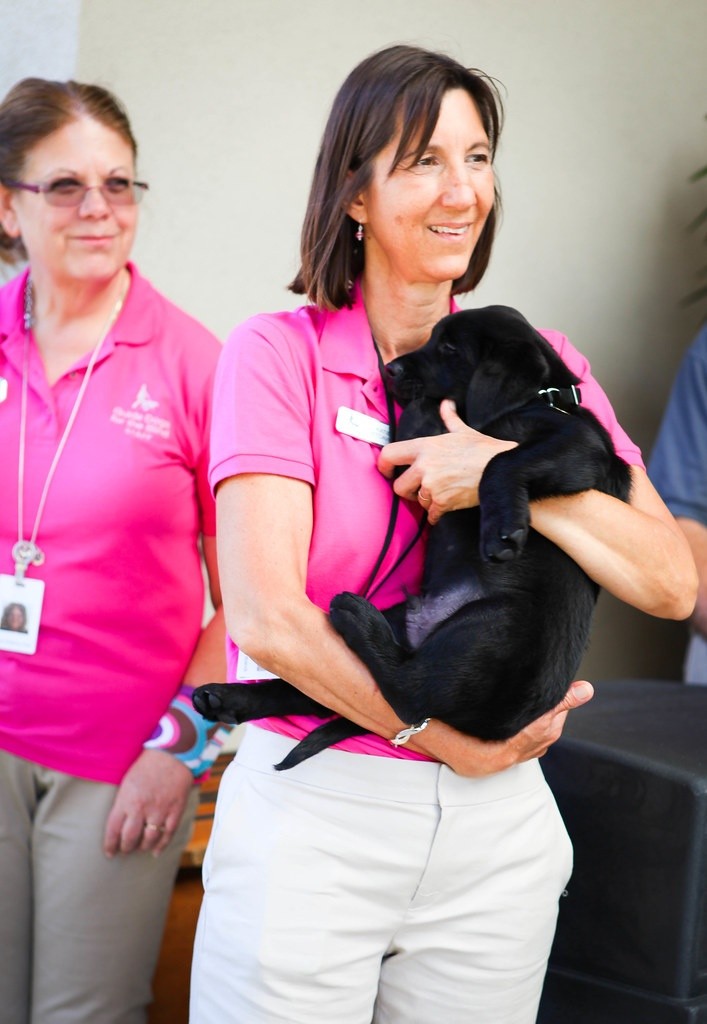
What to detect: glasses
<box><xmin>3</xmin><ymin>177</ymin><xmax>149</xmax><ymax>207</ymax></box>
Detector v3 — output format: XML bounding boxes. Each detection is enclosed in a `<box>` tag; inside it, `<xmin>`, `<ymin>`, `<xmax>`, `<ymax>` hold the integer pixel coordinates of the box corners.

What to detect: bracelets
<box><xmin>390</xmin><ymin>718</ymin><xmax>431</xmax><ymax>748</ymax></box>
<box><xmin>143</xmin><ymin>683</ymin><xmax>237</xmax><ymax>787</ymax></box>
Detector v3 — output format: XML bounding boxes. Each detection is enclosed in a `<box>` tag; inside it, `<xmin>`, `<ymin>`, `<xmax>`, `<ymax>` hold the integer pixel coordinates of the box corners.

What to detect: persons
<box><xmin>648</xmin><ymin>323</ymin><xmax>707</xmax><ymax>684</ymax></box>
<box><xmin>0</xmin><ymin>76</ymin><xmax>239</xmax><ymax>1024</ymax></box>
<box><xmin>165</xmin><ymin>46</ymin><xmax>699</xmax><ymax>1024</ymax></box>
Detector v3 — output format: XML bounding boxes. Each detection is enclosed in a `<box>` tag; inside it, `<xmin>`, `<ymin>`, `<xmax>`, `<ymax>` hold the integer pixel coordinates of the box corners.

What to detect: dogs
<box><xmin>191</xmin><ymin>305</ymin><xmax>634</xmax><ymax>772</ymax></box>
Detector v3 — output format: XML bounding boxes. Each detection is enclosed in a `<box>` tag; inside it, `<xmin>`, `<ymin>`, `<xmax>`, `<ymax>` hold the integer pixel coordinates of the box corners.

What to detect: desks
<box><xmin>541</xmin><ymin>680</ymin><xmax>707</xmax><ymax>1024</ymax></box>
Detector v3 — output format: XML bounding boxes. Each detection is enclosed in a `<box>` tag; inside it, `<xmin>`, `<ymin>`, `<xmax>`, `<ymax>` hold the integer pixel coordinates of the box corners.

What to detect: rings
<box><xmin>143</xmin><ymin>821</ymin><xmax>165</xmax><ymax>832</ymax></box>
<box><xmin>418</xmin><ymin>490</ymin><xmax>430</xmax><ymax>502</ymax></box>
